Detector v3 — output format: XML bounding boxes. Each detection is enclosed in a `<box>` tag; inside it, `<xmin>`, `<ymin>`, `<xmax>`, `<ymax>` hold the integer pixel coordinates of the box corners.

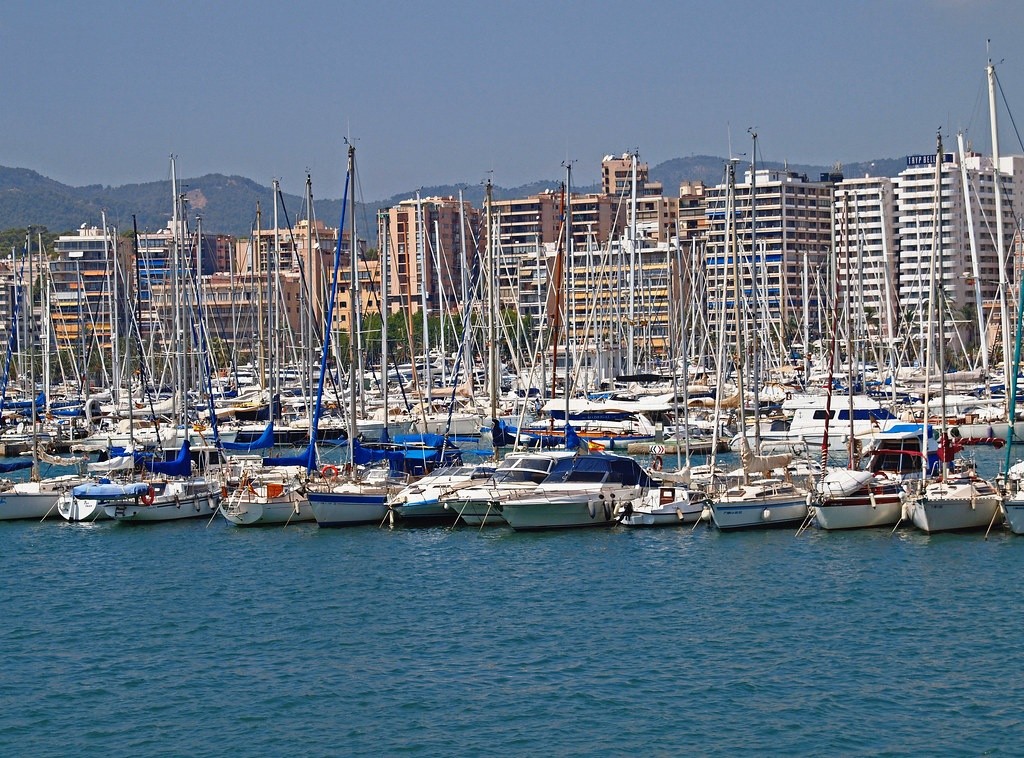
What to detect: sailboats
<box><xmin>0</xmin><ymin>34</ymin><xmax>1024</xmax><ymax>539</ymax></box>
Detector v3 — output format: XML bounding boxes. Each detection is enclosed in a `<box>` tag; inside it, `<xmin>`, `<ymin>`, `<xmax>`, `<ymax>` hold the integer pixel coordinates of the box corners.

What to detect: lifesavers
<box><xmin>652</xmin><ymin>456</ymin><xmax>663</xmax><ymax>471</ymax></box>
<box><xmin>322</xmin><ymin>465</ymin><xmax>338</xmax><ymax>478</ymax></box>
<box><xmin>873</xmin><ymin>472</ymin><xmax>888</xmax><ymax>479</ymax></box>
<box><xmin>141</xmin><ymin>486</ymin><xmax>155</xmax><ymax>506</ymax></box>
